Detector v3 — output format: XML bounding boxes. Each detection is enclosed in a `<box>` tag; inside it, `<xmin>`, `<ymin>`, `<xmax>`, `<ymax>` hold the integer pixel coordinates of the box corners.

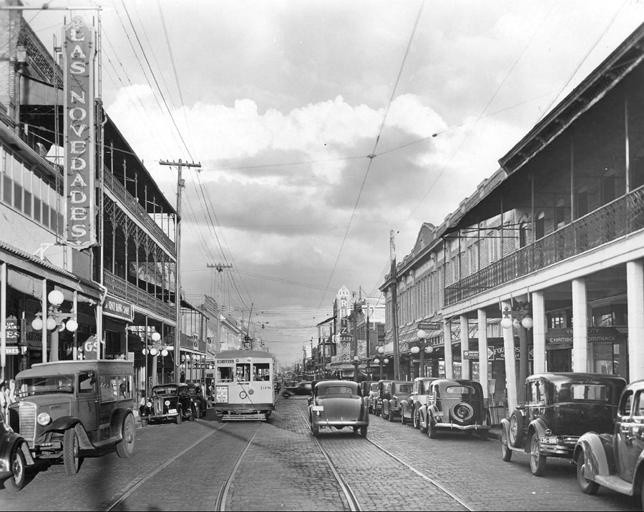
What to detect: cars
<box><xmin>172</xmin><ymin>385</ymin><xmax>206</xmax><ymax>418</ymax></box>
<box><xmin>136</xmin><ymin>381</ymin><xmax>198</xmax><ymax>427</ymax></box>
<box><xmin>0</xmin><ymin>410</ymin><xmax>36</xmax><ymax>494</ymax></box>
<box><xmin>274</xmin><ymin>373</ymin><xmax>491</xmax><ymax>442</ymax></box>
<box><xmin>498</xmin><ymin>369</ymin><xmax>627</xmax><ymax>476</ymax></box>
<box><xmin>569</xmin><ymin>375</ymin><xmax>644</xmax><ymax>511</ymax></box>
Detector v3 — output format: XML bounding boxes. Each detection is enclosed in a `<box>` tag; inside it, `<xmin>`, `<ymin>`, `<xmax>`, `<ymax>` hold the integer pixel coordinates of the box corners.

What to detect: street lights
<box><xmin>348</xmin><ymin>329</ymin><xmax>434</xmax><ymax>384</ymax></box>
<box><xmin>140</xmin><ymin>329</ymin><xmax>206</xmax><ymax>399</ymax></box>
<box><xmin>499</xmin><ymin>300</ymin><xmax>536</xmax><ymax>412</ymax></box>
<box><xmin>30</xmin><ymin>290</ymin><xmax>78</xmax><ymax>365</ymax></box>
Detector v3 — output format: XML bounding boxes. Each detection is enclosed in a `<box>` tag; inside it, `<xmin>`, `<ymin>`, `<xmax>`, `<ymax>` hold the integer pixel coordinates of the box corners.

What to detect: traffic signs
<box><xmin>462</xmin><ymin>346</ymin><xmax>495</xmax><ymax>362</ymax></box>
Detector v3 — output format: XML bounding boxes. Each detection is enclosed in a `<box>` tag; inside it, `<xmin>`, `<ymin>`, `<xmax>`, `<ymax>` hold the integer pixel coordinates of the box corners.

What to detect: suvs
<box><xmin>8</xmin><ymin>355</ymin><xmax>140</xmax><ymax>475</ymax></box>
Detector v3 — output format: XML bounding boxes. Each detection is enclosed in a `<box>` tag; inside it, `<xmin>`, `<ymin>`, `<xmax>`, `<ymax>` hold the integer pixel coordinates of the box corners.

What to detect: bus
<box><xmin>211</xmin><ymin>347</ymin><xmax>281</xmax><ymax>423</ymax></box>
